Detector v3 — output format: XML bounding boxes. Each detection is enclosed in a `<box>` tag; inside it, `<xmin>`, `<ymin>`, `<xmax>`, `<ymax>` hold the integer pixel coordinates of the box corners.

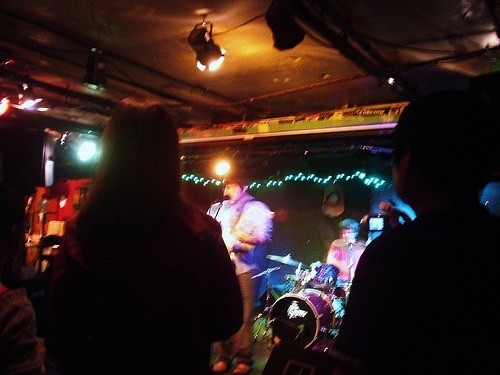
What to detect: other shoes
<box><xmin>233</xmin><ymin>363</ymin><xmax>250</xmax><ymax>374</ymax></box>
<box><xmin>213</xmin><ymin>360</ymin><xmax>228</xmax><ymax>373</ymax></box>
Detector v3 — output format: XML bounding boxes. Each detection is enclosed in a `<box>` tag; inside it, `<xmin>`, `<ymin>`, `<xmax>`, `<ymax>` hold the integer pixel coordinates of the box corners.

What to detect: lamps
<box><xmin>82</xmin><ymin>49</ymin><xmax>109</xmax><ymax>92</ymax></box>
<box><xmin>15</xmin><ymin>79</ymin><xmax>39</xmax><ymax>109</ymax></box>
<box><xmin>264</xmin><ymin>0</ymin><xmax>305</xmax><ymax>51</ymax></box>
<box><xmin>187</xmin><ymin>14</ymin><xmax>225</xmax><ymax>70</ymax></box>
<box><xmin>57</xmin><ymin>134</ymin><xmax>97</xmax><ymax>162</ymax></box>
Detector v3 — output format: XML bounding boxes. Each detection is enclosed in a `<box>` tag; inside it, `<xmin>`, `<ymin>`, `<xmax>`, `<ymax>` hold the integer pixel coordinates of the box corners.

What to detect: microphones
<box><xmin>379</xmin><ymin>202</ymin><xmax>404</xmax><ymax>216</ymax></box>
<box><xmin>212</xmin><ymin>195</ymin><xmax>230</xmax><ymax>205</ymax></box>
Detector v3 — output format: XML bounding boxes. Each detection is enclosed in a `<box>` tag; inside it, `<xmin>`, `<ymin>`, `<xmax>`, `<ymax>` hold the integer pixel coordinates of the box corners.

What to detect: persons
<box><xmin>326</xmin><ymin>219</ymin><xmax>367</xmax><ymax>288</ymax></box>
<box><xmin>206</xmin><ymin>173</ymin><xmax>273</xmax><ymax>375</ymax></box>
<box><xmin>41</xmin><ymin>97</ymin><xmax>244</xmax><ymax>375</ymax></box>
<box><xmin>329</xmin><ymin>91</ymin><xmax>500</xmax><ymax>375</ymax></box>
<box><xmin>0</xmin><ymin>182</ymin><xmax>46</xmax><ymax>375</ymax></box>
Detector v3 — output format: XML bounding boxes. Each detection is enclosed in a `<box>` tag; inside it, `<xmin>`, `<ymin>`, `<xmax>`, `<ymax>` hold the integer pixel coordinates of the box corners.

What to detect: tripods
<box><xmin>250</xmin><ymin>264</ymin><xmax>288</xmax><ymax>342</ymax></box>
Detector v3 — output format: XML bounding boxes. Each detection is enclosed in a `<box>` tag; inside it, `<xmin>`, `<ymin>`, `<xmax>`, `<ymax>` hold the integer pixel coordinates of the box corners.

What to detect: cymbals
<box><xmin>250</xmin><ymin>266</ymin><xmax>280</xmax><ymax>280</ymax></box>
<box><xmin>266</xmin><ymin>255</ymin><xmax>305</xmax><ymax>268</ymax></box>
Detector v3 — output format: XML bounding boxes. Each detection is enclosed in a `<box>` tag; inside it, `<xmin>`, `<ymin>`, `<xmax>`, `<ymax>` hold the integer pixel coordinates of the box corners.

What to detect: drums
<box><xmin>266</xmin><ymin>289</ymin><xmax>335</xmax><ymax>349</ymax></box>
<box><xmin>330</xmin><ymin>279</ymin><xmax>350</xmax><ymax>298</ymax></box>
<box><xmin>303</xmin><ymin>262</ymin><xmax>340</xmax><ymax>291</ymax></box>
